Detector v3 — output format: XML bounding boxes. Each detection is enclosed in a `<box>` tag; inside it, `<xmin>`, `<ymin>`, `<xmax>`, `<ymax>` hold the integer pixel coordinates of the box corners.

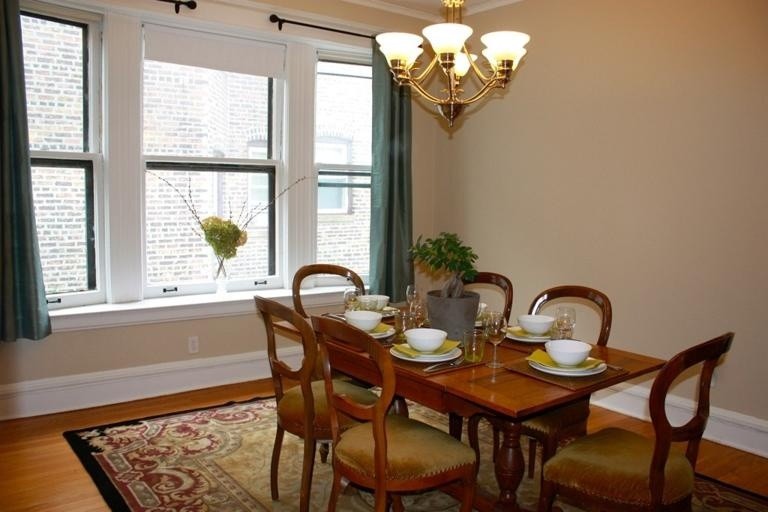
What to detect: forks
<box><xmin>609</xmin><ymin>364</ymin><xmax>630</xmax><ymax>376</ymax></box>
<box><xmin>424</xmin><ymin>356</ymin><xmax>465</xmax><ymax>373</ymax></box>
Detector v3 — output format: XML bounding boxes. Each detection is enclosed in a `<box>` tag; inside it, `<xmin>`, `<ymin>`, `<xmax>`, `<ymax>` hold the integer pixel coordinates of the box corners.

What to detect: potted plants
<box><xmin>144</xmin><ymin>170</ymin><xmax>306</xmax><ymax>295</ymax></box>
<box><xmin>407</xmin><ymin>231</ymin><xmax>480</xmax><ymax>342</ymax></box>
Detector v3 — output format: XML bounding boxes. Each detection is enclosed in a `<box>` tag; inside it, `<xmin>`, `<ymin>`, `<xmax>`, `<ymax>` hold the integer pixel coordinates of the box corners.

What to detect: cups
<box><xmin>554</xmin><ymin>306</ymin><xmax>577</xmax><ymax>339</ymax></box>
<box><xmin>358</xmin><ymin>296</ymin><xmax>377</xmax><ymax>310</ymax></box>
<box><xmin>396</xmin><ymin>283</ymin><xmax>428</xmax><ymax>330</ymax></box>
<box><xmin>342</xmin><ymin>287</ymin><xmax>364</xmax><ymax>310</ymax></box>
<box><xmin>463</xmin><ymin>330</ymin><xmax>486</xmax><ymax>363</ymax></box>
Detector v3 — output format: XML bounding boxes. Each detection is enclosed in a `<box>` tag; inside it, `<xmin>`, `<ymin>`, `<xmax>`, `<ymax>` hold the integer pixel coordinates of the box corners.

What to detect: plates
<box><xmin>526</xmin><ymin>356</ymin><xmax>606</xmax><ymax>376</ymax></box>
<box><xmin>379</xmin><ymin>305</ymin><xmax>400</xmax><ymax>317</ymax></box>
<box><xmin>504</xmin><ymin>326</ymin><xmax>550</xmax><ymax>343</ymax></box>
<box><xmin>475</xmin><ymin>320</ymin><xmax>483</xmax><ymax>327</ymax></box>
<box><xmin>389</xmin><ymin>344</ymin><xmax>461</xmax><ymax>362</ymax></box>
<box><xmin>369</xmin><ymin>322</ymin><xmax>396</xmax><ymax>340</ymax></box>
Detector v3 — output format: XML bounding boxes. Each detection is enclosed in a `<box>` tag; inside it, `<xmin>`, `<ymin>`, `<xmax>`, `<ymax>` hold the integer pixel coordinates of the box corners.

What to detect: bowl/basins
<box><xmin>343</xmin><ymin>310</ymin><xmax>381</xmax><ymax>330</ymax></box>
<box><xmin>402</xmin><ymin>325</ymin><xmax>448</xmax><ymax>354</ymax></box>
<box><xmin>543</xmin><ymin>338</ymin><xmax>592</xmax><ymax>366</ymax></box>
<box><xmin>475</xmin><ymin>303</ymin><xmax>488</xmax><ymax>318</ymax></box>
<box><xmin>516</xmin><ymin>314</ymin><xmax>555</xmax><ymax>334</ymax></box>
<box><xmin>356</xmin><ymin>295</ymin><xmax>390</xmax><ymax>310</ymax></box>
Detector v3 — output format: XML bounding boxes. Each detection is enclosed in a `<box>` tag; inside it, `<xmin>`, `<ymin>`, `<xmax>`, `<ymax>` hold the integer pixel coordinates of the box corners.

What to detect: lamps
<box><xmin>375</xmin><ymin>0</ymin><xmax>530</xmax><ymax>129</ymax></box>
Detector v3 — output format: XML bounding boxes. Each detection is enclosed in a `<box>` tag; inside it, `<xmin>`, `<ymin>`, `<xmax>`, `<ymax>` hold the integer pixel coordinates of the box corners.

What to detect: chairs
<box><xmin>468</xmin><ymin>285</ymin><xmax>612</xmax><ymax>478</ymax></box>
<box><xmin>252</xmin><ymin>296</ymin><xmax>379</xmax><ymax>512</ymax></box>
<box><xmin>293</xmin><ymin>264</ymin><xmax>365</xmax><ymax>462</ymax></box>
<box><xmin>311</xmin><ymin>315</ymin><xmax>477</xmax><ymax>512</ymax></box>
<box><xmin>539</xmin><ymin>332</ymin><xmax>734</xmax><ymax>512</ymax></box>
<box><xmin>395</xmin><ymin>272</ymin><xmax>513</xmax><ymax>444</ymax></box>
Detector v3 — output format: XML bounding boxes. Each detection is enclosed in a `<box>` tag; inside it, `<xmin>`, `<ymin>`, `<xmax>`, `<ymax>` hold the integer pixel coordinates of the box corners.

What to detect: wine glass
<box><xmin>482</xmin><ymin>312</ymin><xmax>508</xmax><ymax>370</ymax></box>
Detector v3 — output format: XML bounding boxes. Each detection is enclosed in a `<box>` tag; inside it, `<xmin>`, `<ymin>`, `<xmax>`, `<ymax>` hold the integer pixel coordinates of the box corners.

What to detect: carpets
<box><xmin>63</xmin><ymin>385</ymin><xmax>768</xmax><ymax>512</ymax></box>
